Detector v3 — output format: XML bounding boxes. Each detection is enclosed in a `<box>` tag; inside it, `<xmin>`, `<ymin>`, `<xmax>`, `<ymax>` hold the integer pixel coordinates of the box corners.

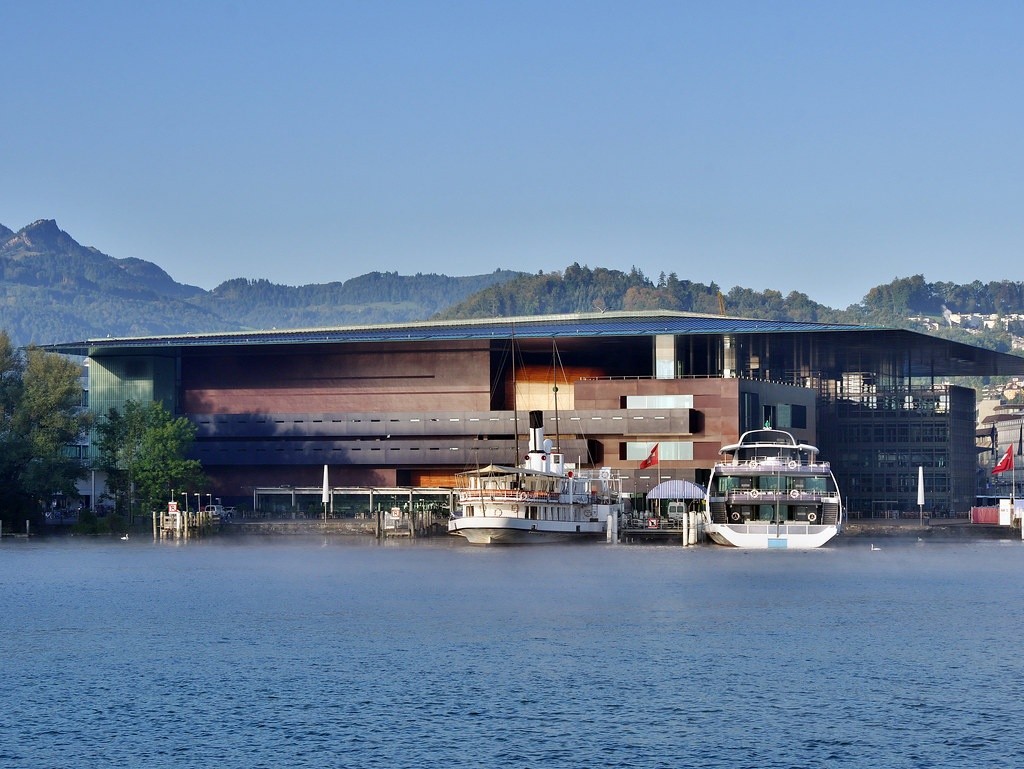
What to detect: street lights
<box><xmin>194</xmin><ymin>494</ymin><xmax>201</xmax><ymax>512</ymax></box>
<box><xmin>182</xmin><ymin>493</ymin><xmax>189</xmax><ymax>512</ymax></box>
<box><xmin>206</xmin><ymin>493</ymin><xmax>211</xmax><ymax>513</ymax></box>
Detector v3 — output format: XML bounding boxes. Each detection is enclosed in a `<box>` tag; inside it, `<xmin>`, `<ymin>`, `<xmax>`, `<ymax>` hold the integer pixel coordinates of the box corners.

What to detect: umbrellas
<box><xmin>917</xmin><ymin>466</ymin><xmax>925</xmax><ymax>527</ymax></box>
<box><xmin>322</xmin><ymin>464</ymin><xmax>330</xmax><ymax>524</ymax></box>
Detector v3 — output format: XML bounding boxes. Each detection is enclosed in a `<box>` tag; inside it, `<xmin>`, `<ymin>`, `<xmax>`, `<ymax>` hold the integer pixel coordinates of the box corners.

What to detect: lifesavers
<box><xmin>520</xmin><ymin>492</ymin><xmax>527</xmax><ymax>501</ymax></box>
<box><xmin>790</xmin><ymin>489</ymin><xmax>799</xmax><ymax>499</ymax></box>
<box><xmin>788</xmin><ymin>460</ymin><xmax>796</xmax><ymax>470</ymax></box>
<box><xmin>748</xmin><ymin>460</ymin><xmax>758</xmax><ymax>470</ymax></box>
<box><xmin>750</xmin><ymin>488</ymin><xmax>759</xmax><ymax>499</ymax></box>
<box><xmin>494</xmin><ymin>507</ymin><xmax>502</xmax><ymax>518</ymax></box>
<box><xmin>731</xmin><ymin>511</ymin><xmax>740</xmax><ymax>520</ymax></box>
<box><xmin>462</xmin><ymin>491</ymin><xmax>468</xmax><ymax>501</ymax></box>
<box><xmin>808</xmin><ymin>512</ymin><xmax>816</xmax><ymax>521</ymax></box>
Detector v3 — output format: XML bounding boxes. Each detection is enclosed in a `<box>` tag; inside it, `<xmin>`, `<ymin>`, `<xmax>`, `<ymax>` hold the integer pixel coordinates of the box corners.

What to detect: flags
<box><xmin>991</xmin><ymin>441</ymin><xmax>1015</xmax><ymax>474</ymax></box>
<box><xmin>639</xmin><ymin>442</ymin><xmax>659</xmax><ymax>470</ymax></box>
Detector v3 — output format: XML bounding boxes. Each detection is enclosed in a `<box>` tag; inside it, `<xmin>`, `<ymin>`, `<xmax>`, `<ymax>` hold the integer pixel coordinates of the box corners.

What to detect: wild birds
<box><xmin>870</xmin><ymin>543</ymin><xmax>882</xmax><ymax>551</ymax></box>
<box><xmin>120</xmin><ymin>533</ymin><xmax>130</xmax><ymax>540</ymax></box>
<box><xmin>917</xmin><ymin>537</ymin><xmax>924</xmax><ymax>542</ymax></box>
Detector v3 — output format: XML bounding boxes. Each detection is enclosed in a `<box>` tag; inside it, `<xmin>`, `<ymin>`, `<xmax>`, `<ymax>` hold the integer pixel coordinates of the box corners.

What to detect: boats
<box><xmin>704</xmin><ymin>405</ymin><xmax>849</xmax><ymax>552</ymax></box>
<box><xmin>447</xmin><ymin>324</ymin><xmax>625</xmax><ymax>548</ymax></box>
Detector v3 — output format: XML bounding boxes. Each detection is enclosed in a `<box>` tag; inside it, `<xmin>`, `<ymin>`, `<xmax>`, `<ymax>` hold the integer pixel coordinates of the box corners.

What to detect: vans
<box><xmin>205</xmin><ymin>505</ymin><xmax>238</xmax><ymax>520</ymax></box>
<box><xmin>668</xmin><ymin>501</ymin><xmax>688</xmax><ymax>524</ymax></box>
